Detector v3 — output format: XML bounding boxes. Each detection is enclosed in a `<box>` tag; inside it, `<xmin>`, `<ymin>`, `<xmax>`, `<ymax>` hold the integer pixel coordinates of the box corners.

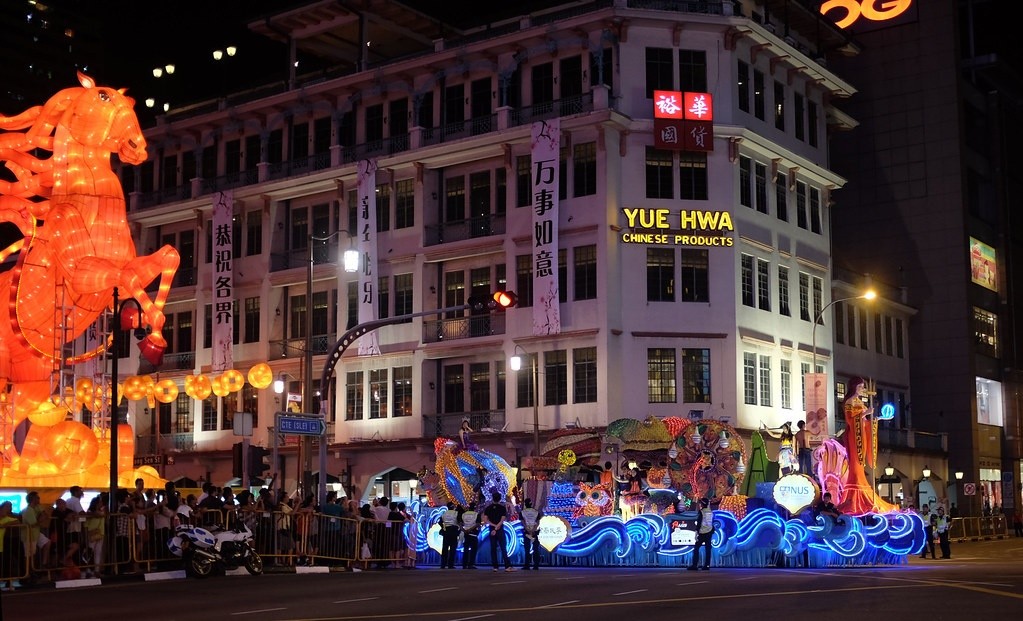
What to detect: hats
<box><xmin>446</xmin><ymin>502</ymin><xmax>455</xmax><ymax>508</ymax></box>
<box><xmin>699</xmin><ymin>498</ymin><xmax>709</xmax><ymax>506</ymax></box>
<box><xmin>469</xmin><ymin>502</ymin><xmax>477</xmax><ymax>508</ymax></box>
<box><xmin>935</xmin><ymin>507</ymin><xmax>945</xmax><ymax>512</ymax></box>
<box><xmin>922</xmin><ymin>503</ymin><xmax>929</xmax><ymax>508</ymax></box>
<box><xmin>524</xmin><ymin>498</ymin><xmax>532</xmax><ymax>505</ymax></box>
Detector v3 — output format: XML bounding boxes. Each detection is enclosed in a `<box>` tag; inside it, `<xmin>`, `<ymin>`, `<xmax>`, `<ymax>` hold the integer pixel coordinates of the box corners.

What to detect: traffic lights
<box><xmin>467</xmin><ymin>290</ymin><xmax>523</xmax><ymax>323</ymax></box>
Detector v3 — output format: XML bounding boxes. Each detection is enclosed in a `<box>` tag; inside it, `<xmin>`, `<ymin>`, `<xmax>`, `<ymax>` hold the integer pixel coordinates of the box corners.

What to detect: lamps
<box><xmin>884</xmin><ymin>462</ymin><xmax>966</xmax><ymax>489</ymax></box>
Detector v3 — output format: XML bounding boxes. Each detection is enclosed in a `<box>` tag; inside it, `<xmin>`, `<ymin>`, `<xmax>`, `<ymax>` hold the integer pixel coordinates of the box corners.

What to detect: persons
<box><xmin>950</xmin><ymin>503</ymin><xmax>957</xmax><ymax>514</ymax></box>
<box><xmin>933</xmin><ymin>507</ymin><xmax>953</xmax><ymax>559</ymax></box>
<box><xmin>687</xmin><ymin>498</ymin><xmax>716</xmax><ymax>570</ymax></box>
<box><xmin>600</xmin><ymin>460</ymin><xmax>613</xmax><ymax>488</ymax></box>
<box><xmin>817</xmin><ymin>493</ymin><xmax>839</xmax><ymax>517</ymax></box>
<box><xmin>1014</xmin><ymin>509</ymin><xmax>1023</xmax><ymax>537</ymax></box>
<box><xmin>484</xmin><ymin>492</ymin><xmax>517</xmax><ymax>572</ymax></box>
<box><xmin>918</xmin><ymin>504</ymin><xmax>936</xmax><ymax>559</ymax></box>
<box><xmin>793</xmin><ymin>420</ymin><xmax>820</xmax><ymax>478</ymax></box>
<box><xmin>983</xmin><ymin>501</ymin><xmax>1002</xmax><ymax>517</ymax></box>
<box><xmin>838</xmin><ymin>377</ymin><xmax>899</xmax><ymax>514</ymax></box>
<box><xmin>438</xmin><ymin>502</ymin><xmax>461</xmax><ymax>570</ymax></box>
<box><xmin>0</xmin><ymin>479</ymin><xmax>414</xmax><ymax>589</ymax></box>
<box><xmin>460</xmin><ymin>502</ymin><xmax>481</xmax><ymax>568</ymax></box>
<box><xmin>619</xmin><ymin>465</ymin><xmax>642</xmax><ymax>521</ymax></box>
<box><xmin>519</xmin><ymin>498</ymin><xmax>541</xmax><ymax>570</ymax></box>
<box><xmin>459</xmin><ymin>420</ymin><xmax>473</xmax><ymax>450</ymax></box>
<box><xmin>764</xmin><ymin>421</ymin><xmax>799</xmax><ymax>475</ymax></box>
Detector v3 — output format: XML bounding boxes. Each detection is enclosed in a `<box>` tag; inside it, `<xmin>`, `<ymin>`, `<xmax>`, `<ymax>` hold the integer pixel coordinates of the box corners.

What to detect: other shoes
<box><xmin>533</xmin><ymin>567</ymin><xmax>539</xmax><ymax>570</ymax></box>
<box><xmin>702</xmin><ymin>564</ymin><xmax>711</xmax><ymax>570</ymax></box>
<box><xmin>940</xmin><ymin>556</ymin><xmax>951</xmax><ymax>559</ymax></box>
<box><xmin>919</xmin><ymin>557</ymin><xmax>926</xmax><ymax>558</ymax></box>
<box><xmin>687</xmin><ymin>565</ymin><xmax>699</xmax><ymax>571</ymax></box>
<box><xmin>448</xmin><ymin>566</ymin><xmax>458</xmax><ymax>569</ymax></box>
<box><xmin>385</xmin><ymin>562</ymin><xmax>403</xmax><ymax>569</ymax></box>
<box><xmin>467</xmin><ymin>565</ymin><xmax>477</xmax><ymax>569</ymax></box>
<box><xmin>494</xmin><ymin>569</ymin><xmax>499</xmax><ymax>572</ymax></box>
<box><xmin>440</xmin><ymin>566</ymin><xmax>446</xmax><ymax>569</ymax></box>
<box><xmin>462</xmin><ymin>566</ymin><xmax>468</xmax><ymax>569</ymax></box>
<box><xmin>521</xmin><ymin>566</ymin><xmax>530</xmax><ymax>570</ymax></box>
<box><xmin>504</xmin><ymin>566</ymin><xmax>518</xmax><ymax>572</ymax></box>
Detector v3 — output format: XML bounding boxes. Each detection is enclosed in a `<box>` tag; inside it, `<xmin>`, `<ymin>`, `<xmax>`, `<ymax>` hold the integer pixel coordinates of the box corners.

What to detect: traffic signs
<box><xmin>277</xmin><ymin>414</ymin><xmax>328</xmax><ymax>437</ymax></box>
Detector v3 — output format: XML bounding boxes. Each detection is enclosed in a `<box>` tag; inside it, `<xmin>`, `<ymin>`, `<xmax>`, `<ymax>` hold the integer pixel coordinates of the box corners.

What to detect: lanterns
<box><xmin>28</xmin><ymin>376</ymin><xmax>178</xmax><ymax>426</ymax></box>
<box><xmin>184</xmin><ymin>363</ymin><xmax>273</xmax><ymax>400</ymax></box>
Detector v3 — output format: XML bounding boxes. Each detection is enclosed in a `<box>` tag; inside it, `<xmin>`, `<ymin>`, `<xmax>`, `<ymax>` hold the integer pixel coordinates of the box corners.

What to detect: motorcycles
<box><xmin>168</xmin><ymin>505</ymin><xmax>264</xmax><ymax>579</ymax></box>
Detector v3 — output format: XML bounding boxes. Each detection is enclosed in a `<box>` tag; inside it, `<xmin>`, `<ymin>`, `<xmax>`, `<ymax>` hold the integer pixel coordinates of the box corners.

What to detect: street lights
<box><xmin>300</xmin><ymin>227</ymin><xmax>361</xmax><ymax>510</ymax></box>
<box><xmin>509</xmin><ymin>344</ymin><xmax>543</xmax><ymax>461</ymax></box>
<box><xmin>107</xmin><ymin>284</ymin><xmax>153</xmax><ymax>584</ymax></box>
<box><xmin>809</xmin><ymin>290</ymin><xmax>875</xmax><ymax>372</ymax></box>
